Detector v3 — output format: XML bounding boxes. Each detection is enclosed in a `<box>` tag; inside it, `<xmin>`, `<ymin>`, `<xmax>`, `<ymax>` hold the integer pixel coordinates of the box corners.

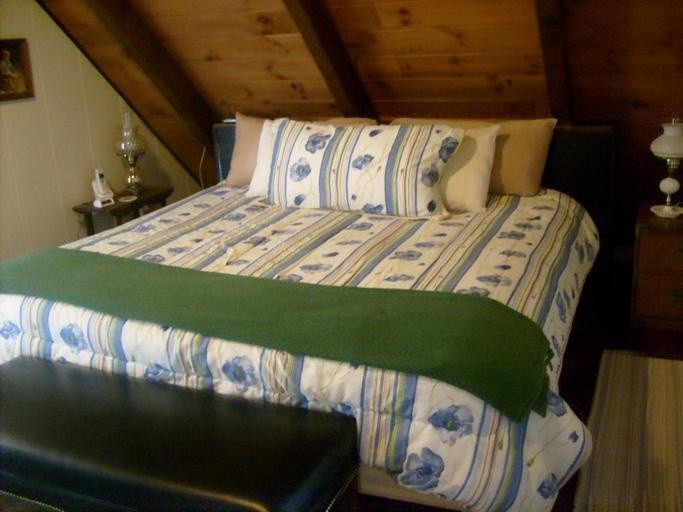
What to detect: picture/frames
<box><xmin>0</xmin><ymin>38</ymin><xmax>35</xmax><ymax>105</ymax></box>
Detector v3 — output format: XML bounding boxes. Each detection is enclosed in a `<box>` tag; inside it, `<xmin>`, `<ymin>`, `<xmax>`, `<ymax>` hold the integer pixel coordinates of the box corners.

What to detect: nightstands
<box><xmin>629</xmin><ymin>195</ymin><xmax>683</xmax><ymax>337</ymax></box>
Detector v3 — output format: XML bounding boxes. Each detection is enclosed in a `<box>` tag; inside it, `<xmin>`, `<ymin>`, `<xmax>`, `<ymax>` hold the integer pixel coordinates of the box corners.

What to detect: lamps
<box><xmin>646</xmin><ymin>112</ymin><xmax>682</xmax><ymax>221</ymax></box>
<box><xmin>108</xmin><ymin>110</ymin><xmax>149</xmax><ymax>199</ymax></box>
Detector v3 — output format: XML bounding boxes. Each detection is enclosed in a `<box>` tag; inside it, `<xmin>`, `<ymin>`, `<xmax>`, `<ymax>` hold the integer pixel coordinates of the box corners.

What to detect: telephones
<box><xmin>92</xmin><ymin>168</ymin><xmax>115</xmax><ymax>208</ymax></box>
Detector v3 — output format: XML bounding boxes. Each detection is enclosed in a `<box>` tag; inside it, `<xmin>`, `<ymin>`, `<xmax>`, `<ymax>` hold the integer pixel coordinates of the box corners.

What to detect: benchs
<box><xmin>0</xmin><ymin>355</ymin><xmax>361</xmax><ymax>512</ymax></box>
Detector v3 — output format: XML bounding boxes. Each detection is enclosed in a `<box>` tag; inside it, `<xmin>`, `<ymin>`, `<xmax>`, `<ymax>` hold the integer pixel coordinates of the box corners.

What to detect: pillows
<box><xmin>245</xmin><ymin>118</ymin><xmax>277</xmax><ymax>199</ymax></box>
<box><xmin>428</xmin><ymin>122</ymin><xmax>500</xmax><ymax>215</ymax></box>
<box><xmin>263</xmin><ymin>114</ymin><xmax>463</xmax><ymax>221</ymax></box>
<box><xmin>385</xmin><ymin>114</ymin><xmax>558</xmax><ymax>199</ymax></box>
<box><xmin>224</xmin><ymin>105</ymin><xmax>378</xmax><ymax>193</ymax></box>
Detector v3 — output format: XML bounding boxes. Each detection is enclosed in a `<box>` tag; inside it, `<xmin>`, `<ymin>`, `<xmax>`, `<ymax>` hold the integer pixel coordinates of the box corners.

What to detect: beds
<box><xmin>0</xmin><ymin>112</ymin><xmax>633</xmax><ymax>510</ymax></box>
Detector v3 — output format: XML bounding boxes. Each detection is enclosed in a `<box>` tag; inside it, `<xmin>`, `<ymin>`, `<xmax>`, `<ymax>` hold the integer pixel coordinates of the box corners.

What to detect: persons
<box><xmin>0</xmin><ymin>47</ymin><xmax>21</xmax><ymax>92</ymax></box>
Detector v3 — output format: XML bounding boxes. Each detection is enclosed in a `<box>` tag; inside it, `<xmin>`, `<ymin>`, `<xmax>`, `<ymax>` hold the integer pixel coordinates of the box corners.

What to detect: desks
<box><xmin>69</xmin><ymin>181</ymin><xmax>175</xmax><ymax>240</ymax></box>
